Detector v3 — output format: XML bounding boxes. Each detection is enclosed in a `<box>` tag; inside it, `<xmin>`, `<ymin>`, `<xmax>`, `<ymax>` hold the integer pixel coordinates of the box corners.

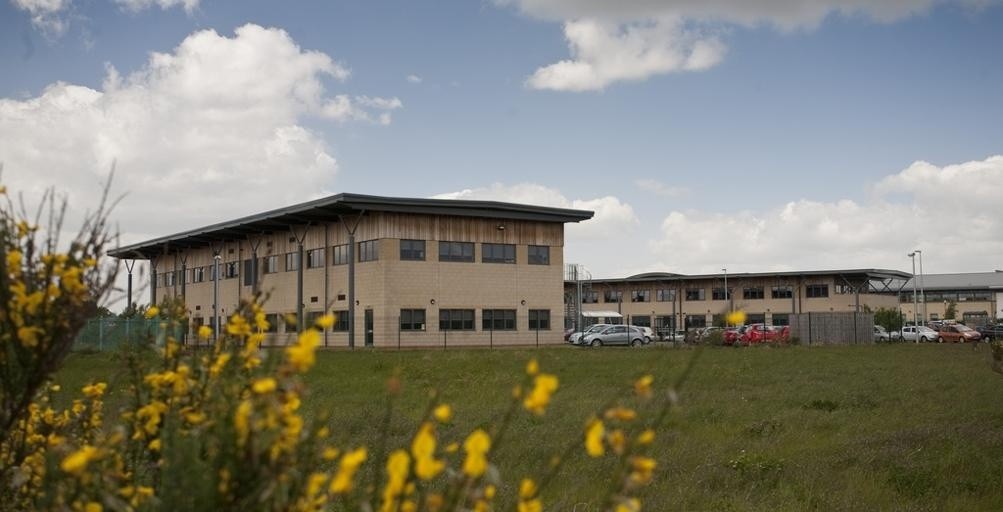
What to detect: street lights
<box><xmin>914</xmin><ymin>249</ymin><xmax>923</xmax><ymax>325</ymax></box>
<box><xmin>907</xmin><ymin>252</ymin><xmax>921</xmax><ymax>345</ymax></box>
<box><xmin>212</xmin><ymin>255</ymin><xmax>221</xmax><ymax>348</ymax></box>
<box><xmin>721</xmin><ymin>268</ymin><xmax>728</xmax><ymax>329</ymax></box>
